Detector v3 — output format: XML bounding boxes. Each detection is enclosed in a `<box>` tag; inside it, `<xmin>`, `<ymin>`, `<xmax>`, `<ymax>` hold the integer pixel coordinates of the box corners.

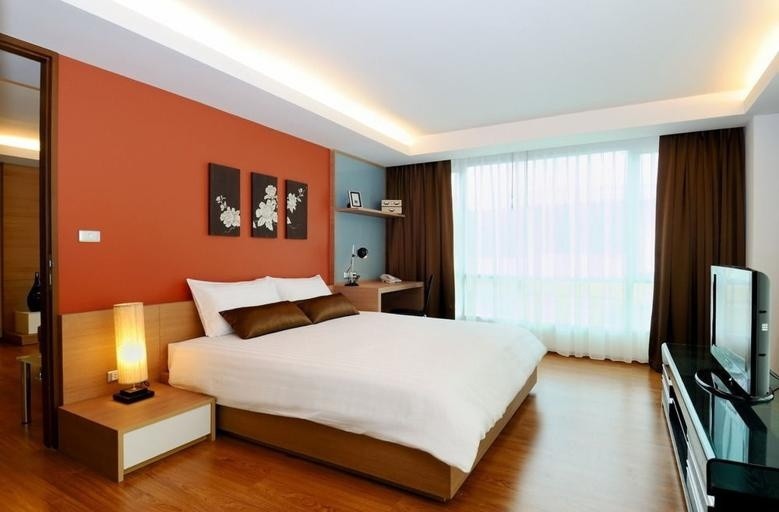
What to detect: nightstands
<box><xmin>57</xmin><ymin>381</ymin><xmax>217</xmax><ymax>482</ymax></box>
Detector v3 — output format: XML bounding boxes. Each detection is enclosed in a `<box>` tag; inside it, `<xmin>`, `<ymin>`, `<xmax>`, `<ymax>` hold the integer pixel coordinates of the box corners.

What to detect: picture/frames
<box><xmin>346</xmin><ymin>190</ymin><xmax>362</xmax><ymax>208</ymax></box>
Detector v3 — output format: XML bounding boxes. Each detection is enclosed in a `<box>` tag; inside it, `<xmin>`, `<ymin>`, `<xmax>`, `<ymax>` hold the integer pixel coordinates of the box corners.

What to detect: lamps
<box><xmin>111</xmin><ymin>300</ymin><xmax>154</xmax><ymax>405</ymax></box>
<box><xmin>343</xmin><ymin>244</ymin><xmax>368</xmax><ymax>287</ymax></box>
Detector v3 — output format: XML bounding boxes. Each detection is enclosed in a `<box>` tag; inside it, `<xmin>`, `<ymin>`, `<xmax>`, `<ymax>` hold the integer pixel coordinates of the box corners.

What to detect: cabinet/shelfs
<box><xmin>659</xmin><ymin>341</ymin><xmax>778</xmax><ymax>511</ymax></box>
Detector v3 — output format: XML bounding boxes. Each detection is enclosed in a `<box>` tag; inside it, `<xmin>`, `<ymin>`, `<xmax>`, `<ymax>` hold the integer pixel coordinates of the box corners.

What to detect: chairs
<box><xmin>388</xmin><ymin>272</ymin><xmax>435</xmax><ymax>317</ymax></box>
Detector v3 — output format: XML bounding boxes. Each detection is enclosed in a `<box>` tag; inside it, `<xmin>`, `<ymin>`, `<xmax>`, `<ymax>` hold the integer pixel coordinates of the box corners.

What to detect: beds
<box><xmin>159</xmin><ymin>311</ymin><xmax>539</xmax><ymax>502</ymax></box>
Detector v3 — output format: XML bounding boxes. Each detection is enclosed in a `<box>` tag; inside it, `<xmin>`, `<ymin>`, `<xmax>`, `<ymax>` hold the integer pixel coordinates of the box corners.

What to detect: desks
<box><xmin>334</xmin><ymin>281</ymin><xmax>424</xmax><ymax>313</ymax></box>
<box><xmin>16</xmin><ymin>353</ymin><xmax>42</xmax><ymax>424</ymax></box>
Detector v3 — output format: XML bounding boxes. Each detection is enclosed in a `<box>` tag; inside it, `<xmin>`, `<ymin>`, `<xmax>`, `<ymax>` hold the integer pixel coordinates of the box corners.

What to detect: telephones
<box><xmin>380</xmin><ymin>273</ymin><xmax>402</xmax><ymax>284</ymax></box>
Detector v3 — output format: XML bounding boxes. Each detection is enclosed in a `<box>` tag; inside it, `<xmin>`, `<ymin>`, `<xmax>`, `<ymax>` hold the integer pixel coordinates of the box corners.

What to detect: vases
<box><xmin>26</xmin><ymin>272</ymin><xmax>41</xmax><ymax>312</ymax></box>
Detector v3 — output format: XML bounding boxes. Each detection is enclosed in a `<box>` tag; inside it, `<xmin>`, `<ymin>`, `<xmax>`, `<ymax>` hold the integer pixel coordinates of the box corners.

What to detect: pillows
<box><xmin>184</xmin><ymin>274</ymin><xmax>362</xmax><ymax>341</ymax></box>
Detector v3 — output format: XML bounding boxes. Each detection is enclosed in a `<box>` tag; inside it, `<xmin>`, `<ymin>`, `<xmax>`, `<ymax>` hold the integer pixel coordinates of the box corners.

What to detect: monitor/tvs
<box><xmin>695</xmin><ymin>265</ymin><xmax>774</xmax><ymax>405</ymax></box>
